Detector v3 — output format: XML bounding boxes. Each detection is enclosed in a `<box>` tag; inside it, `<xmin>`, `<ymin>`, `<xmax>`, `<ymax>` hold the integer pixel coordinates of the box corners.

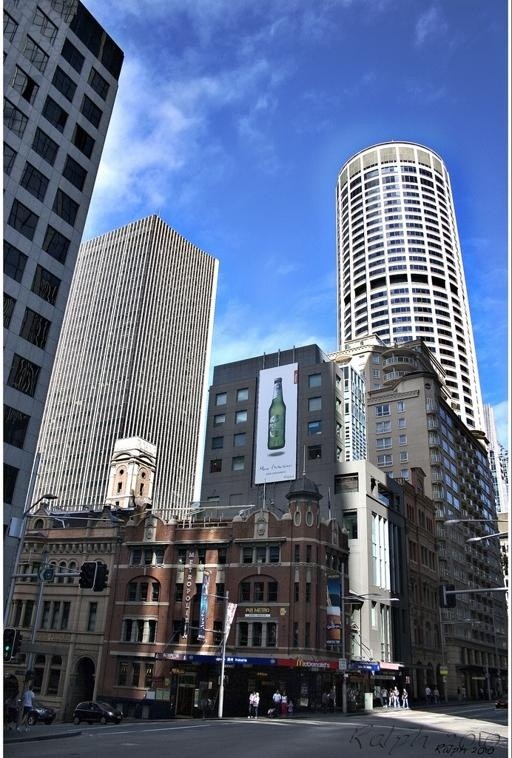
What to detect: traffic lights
<box><xmin>94</xmin><ymin>561</ymin><xmax>109</xmax><ymax>592</ymax></box>
<box><xmin>3</xmin><ymin>628</ymin><xmax>17</xmax><ymax>662</ymax></box>
<box><xmin>79</xmin><ymin>561</ymin><xmax>97</xmax><ymax>590</ymax></box>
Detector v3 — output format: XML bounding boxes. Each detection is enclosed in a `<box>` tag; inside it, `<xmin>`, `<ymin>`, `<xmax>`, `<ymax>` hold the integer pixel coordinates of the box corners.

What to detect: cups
<box><xmin>327</xmin><ymin>607</ymin><xmax>340</xmax><ymax>644</ymax></box>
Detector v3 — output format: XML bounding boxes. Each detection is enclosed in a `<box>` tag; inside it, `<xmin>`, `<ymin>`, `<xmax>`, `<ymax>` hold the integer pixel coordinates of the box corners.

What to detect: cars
<box><xmin>8</xmin><ymin>697</ymin><xmax>56</xmax><ymax>726</ymax></box>
<box><xmin>73</xmin><ymin>700</ymin><xmax>123</xmax><ymax>725</ymax></box>
<box><xmin>494</xmin><ymin>694</ymin><xmax>507</xmax><ymax>709</ymax></box>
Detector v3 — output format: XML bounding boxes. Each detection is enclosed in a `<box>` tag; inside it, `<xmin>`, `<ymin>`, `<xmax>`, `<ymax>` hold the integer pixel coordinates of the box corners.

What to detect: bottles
<box><xmin>268</xmin><ymin>377</ymin><xmax>285</xmax><ymax>449</ymax></box>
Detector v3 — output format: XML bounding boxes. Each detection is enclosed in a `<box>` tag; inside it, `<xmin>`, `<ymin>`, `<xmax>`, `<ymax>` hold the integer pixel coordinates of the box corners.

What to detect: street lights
<box><xmin>438</xmin><ymin>605</ymin><xmax>481</xmax><ymax>704</ymax></box>
<box><xmin>4</xmin><ymin>453</ymin><xmax>125</xmax><ymax>634</ymax></box>
<box><xmin>443</xmin><ymin>517</ymin><xmax>509</xmax><ymax>543</ymax></box>
<box><xmin>341</xmin><ymin>562</ymin><xmax>400</xmax><ymax>714</ymax></box>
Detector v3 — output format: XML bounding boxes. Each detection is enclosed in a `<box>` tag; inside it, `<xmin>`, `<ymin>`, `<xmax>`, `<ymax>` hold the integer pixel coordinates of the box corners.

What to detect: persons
<box><xmin>8</xmin><ymin>693</ymin><xmax>18</xmax><ymax>731</ymax></box>
<box><xmin>247</xmin><ymin>685</ymin><xmax>493</xmax><ymax>719</ymax></box>
<box><xmin>17</xmin><ymin>686</ymin><xmax>35</xmax><ymax>733</ymax></box>
